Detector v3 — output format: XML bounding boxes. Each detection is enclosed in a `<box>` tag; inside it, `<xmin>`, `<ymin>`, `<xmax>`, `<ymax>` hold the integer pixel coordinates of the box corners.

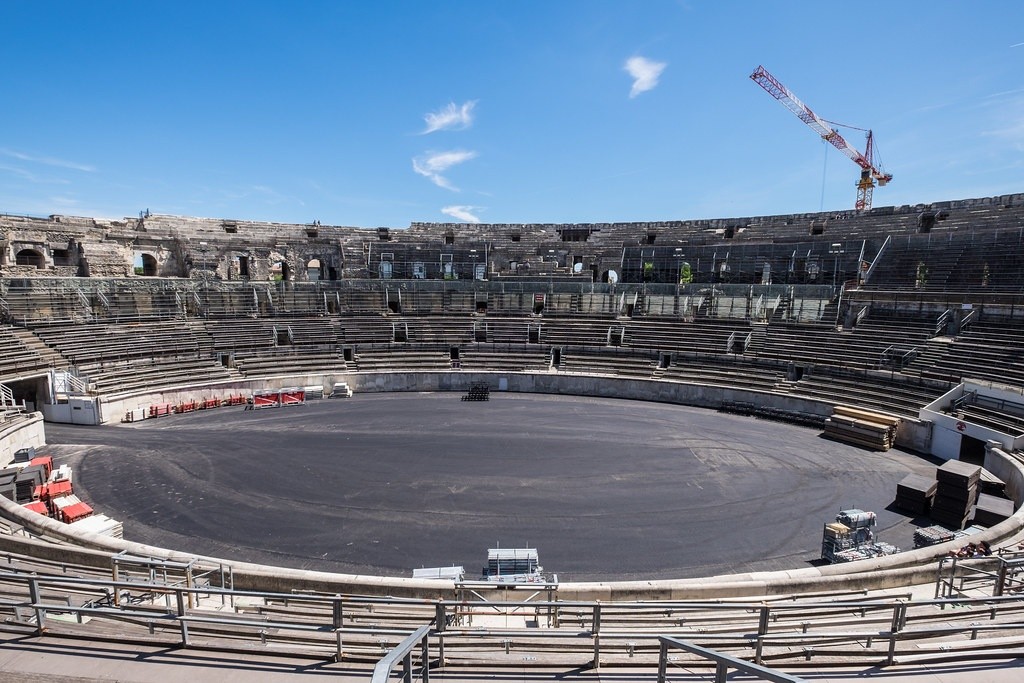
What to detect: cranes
<box><xmin>749</xmin><ymin>65</ymin><xmax>892</xmax><ymax>211</ymax></box>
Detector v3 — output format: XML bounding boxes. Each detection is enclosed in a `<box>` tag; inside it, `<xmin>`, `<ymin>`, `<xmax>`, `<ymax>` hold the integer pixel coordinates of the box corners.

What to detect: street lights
<box><xmin>199</xmin><ymin>240</ymin><xmax>212</xmax><ymax>321</ymax></box>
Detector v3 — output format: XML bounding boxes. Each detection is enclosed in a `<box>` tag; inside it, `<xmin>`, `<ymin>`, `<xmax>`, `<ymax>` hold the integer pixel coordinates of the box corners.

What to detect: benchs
<box><xmin>0</xmin><ymin>515</ymin><xmax>1023</xmax><ymax>679</ymax></box>
<box><xmin>1</xmin><ymin>314</ymin><xmax>1024</xmax><ymax>449</ymax></box>
